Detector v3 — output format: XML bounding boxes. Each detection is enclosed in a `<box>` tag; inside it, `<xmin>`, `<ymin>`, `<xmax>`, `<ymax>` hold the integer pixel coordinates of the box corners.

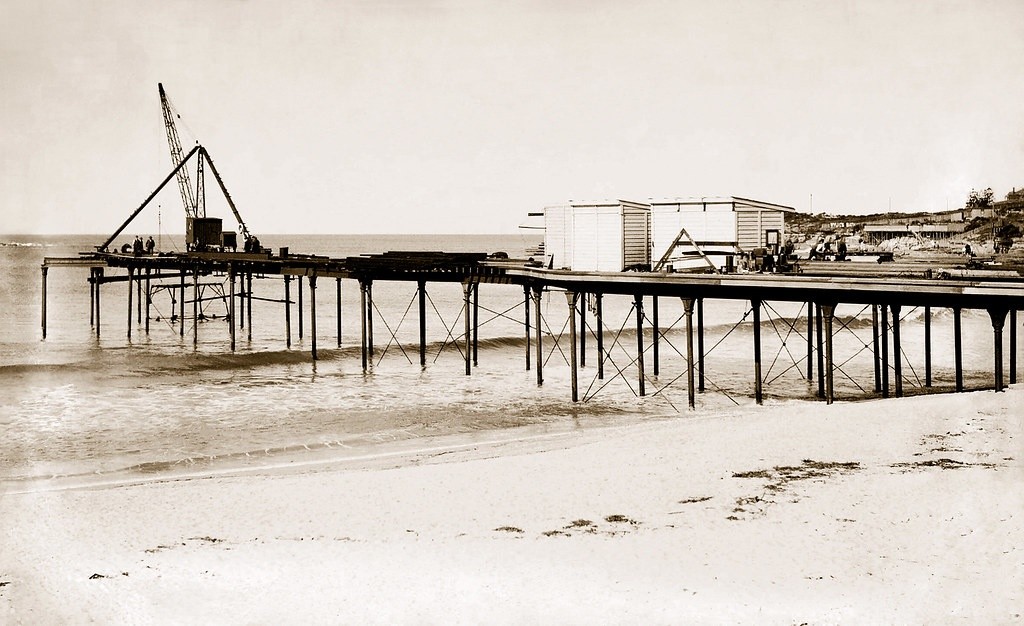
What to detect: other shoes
<box><xmin>813</xmin><ymin>257</ymin><xmax>818</xmax><ymax>261</ymax></box>
<box><xmin>757</xmin><ymin>269</ymin><xmax>764</xmax><ymax>274</ymax></box>
<box><xmin>805</xmin><ymin>258</ymin><xmax>811</xmax><ymax>261</ymax></box>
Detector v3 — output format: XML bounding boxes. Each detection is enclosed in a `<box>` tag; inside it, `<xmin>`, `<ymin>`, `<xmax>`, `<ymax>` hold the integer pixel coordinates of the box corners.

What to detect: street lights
<box><xmin>810</xmin><ymin>194</ymin><xmax>812</xmax><ymax>213</ymax></box>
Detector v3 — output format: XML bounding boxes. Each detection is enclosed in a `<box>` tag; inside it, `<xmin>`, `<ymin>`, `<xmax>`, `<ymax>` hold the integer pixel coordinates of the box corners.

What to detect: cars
<box><xmin>492</xmin><ymin>252</ymin><xmax>508</xmax><ymax>259</ymax></box>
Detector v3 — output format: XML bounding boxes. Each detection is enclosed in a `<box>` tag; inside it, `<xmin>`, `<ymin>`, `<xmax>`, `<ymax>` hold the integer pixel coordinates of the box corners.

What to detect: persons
<box><xmin>738</xmin><ymin>239</ymin><xmax>799</xmax><ymax>275</ymax></box>
<box><xmin>242</xmin><ymin>236</ymin><xmax>261</xmax><ymax>253</ymax></box>
<box><xmin>808</xmin><ymin>235</ymin><xmax>847</xmax><ymax>262</ymax></box>
<box><xmin>133</xmin><ymin>235</ymin><xmax>142</xmax><ymax>257</ymax></box>
<box><xmin>145</xmin><ymin>237</ymin><xmax>155</xmax><ymax>256</ymax></box>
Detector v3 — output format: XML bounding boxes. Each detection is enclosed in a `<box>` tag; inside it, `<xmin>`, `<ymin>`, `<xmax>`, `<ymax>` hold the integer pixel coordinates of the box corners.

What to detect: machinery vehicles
<box><xmin>158</xmin><ymin>82</ymin><xmax>237</xmax><ymax>252</ymax></box>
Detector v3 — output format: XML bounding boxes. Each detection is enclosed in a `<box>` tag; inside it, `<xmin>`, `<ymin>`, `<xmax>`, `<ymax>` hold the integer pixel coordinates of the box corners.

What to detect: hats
<box><xmin>148</xmin><ymin>236</ymin><xmax>153</xmax><ymax>238</ymax></box>
<box><xmin>139</xmin><ymin>237</ymin><xmax>143</xmax><ymax>239</ymax></box>
<box><xmin>787</xmin><ymin>239</ymin><xmax>793</xmax><ymax>244</ymax></box>
